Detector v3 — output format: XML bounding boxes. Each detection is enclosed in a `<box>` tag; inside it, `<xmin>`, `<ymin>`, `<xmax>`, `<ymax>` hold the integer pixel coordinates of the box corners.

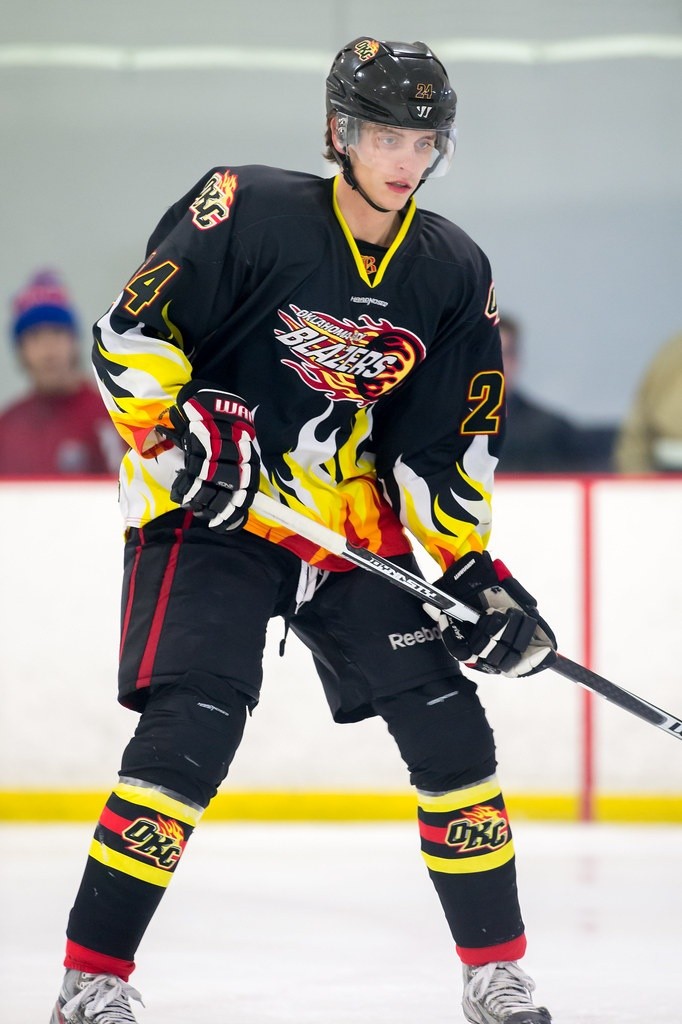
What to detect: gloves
<box><xmin>167</xmin><ymin>389</ymin><xmax>262</xmax><ymax>536</ymax></box>
<box><xmin>416</xmin><ymin>549</ymin><xmax>558</xmax><ymax>678</ymax></box>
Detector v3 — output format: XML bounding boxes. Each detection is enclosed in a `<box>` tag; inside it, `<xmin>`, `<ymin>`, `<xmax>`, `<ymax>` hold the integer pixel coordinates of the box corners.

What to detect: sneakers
<box><xmin>49</xmin><ymin>970</ymin><xmax>146</xmax><ymax>1024</ymax></box>
<box><xmin>461</xmin><ymin>961</ymin><xmax>552</xmax><ymax>1024</ymax></box>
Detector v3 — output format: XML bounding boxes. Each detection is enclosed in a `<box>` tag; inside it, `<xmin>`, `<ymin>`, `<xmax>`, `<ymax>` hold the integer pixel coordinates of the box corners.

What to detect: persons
<box><xmin>49</xmin><ymin>36</ymin><xmax>569</xmax><ymax>1024</ymax></box>
<box><xmin>0</xmin><ymin>269</ymin><xmax>681</xmax><ymax>477</ymax></box>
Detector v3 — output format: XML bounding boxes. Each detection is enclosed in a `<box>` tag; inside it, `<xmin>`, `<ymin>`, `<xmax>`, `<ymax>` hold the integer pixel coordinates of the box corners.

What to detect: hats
<box><xmin>10</xmin><ymin>273</ymin><xmax>77</xmax><ymax>335</ymax></box>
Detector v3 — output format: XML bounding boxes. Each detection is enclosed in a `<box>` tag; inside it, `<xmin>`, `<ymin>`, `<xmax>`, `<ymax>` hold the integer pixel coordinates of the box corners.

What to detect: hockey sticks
<box><xmin>232</xmin><ymin>486</ymin><xmax>682</xmax><ymax>738</ymax></box>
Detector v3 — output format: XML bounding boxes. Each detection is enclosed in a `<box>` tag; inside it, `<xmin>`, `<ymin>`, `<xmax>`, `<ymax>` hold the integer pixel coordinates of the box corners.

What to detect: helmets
<box><xmin>325</xmin><ymin>36</ymin><xmax>458</xmax><ymax>130</ymax></box>
